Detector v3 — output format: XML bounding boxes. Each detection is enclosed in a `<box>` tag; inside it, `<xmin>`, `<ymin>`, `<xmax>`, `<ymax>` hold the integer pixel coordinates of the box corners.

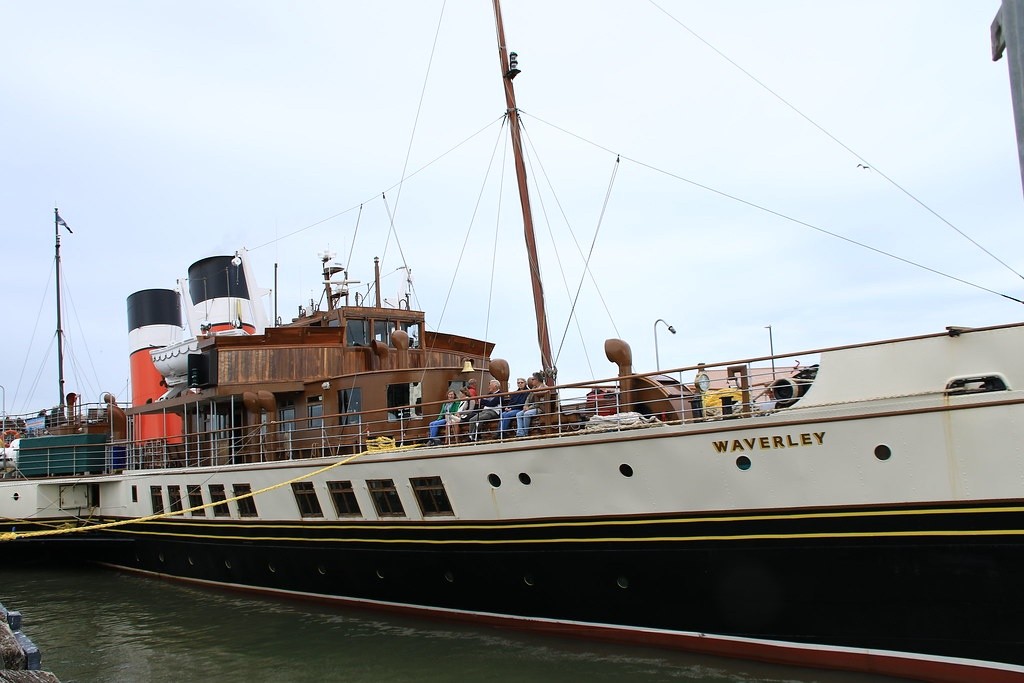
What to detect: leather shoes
<box><xmin>491</xmin><ymin>432</ymin><xmax>500</xmax><ymax>439</ymax></box>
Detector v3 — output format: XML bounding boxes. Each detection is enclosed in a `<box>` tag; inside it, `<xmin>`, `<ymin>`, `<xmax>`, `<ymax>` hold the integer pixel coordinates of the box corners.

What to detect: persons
<box><xmin>424</xmin><ymin>373</ymin><xmax>551</xmax><ymax>446</ymax></box>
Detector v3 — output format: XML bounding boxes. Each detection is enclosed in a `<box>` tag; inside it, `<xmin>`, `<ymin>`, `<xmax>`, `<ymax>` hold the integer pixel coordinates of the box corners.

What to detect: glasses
<box><xmin>532</xmin><ymin>378</ymin><xmax>537</xmax><ymax>380</ymax></box>
<box><xmin>517</xmin><ymin>382</ymin><xmax>525</xmax><ymax>385</ymax></box>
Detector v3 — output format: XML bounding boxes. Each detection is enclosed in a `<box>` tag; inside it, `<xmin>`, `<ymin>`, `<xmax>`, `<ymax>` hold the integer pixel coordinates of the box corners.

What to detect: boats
<box><xmin>0</xmin><ymin>0</ymin><xmax>1024</xmax><ymax>683</ymax></box>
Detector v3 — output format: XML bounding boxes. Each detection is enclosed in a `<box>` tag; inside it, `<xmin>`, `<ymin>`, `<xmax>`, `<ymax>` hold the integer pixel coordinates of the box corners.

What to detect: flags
<box><xmin>58</xmin><ymin>215</ymin><xmax>73</xmax><ymax>233</ymax></box>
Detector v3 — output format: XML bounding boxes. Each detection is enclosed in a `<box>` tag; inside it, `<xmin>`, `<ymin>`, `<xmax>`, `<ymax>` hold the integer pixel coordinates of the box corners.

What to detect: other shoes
<box><xmin>463</xmin><ymin>432</ymin><xmax>469</xmax><ymax>441</ymax></box>
<box><xmin>425</xmin><ymin>440</ymin><xmax>434</xmax><ymax>446</ymax></box>
